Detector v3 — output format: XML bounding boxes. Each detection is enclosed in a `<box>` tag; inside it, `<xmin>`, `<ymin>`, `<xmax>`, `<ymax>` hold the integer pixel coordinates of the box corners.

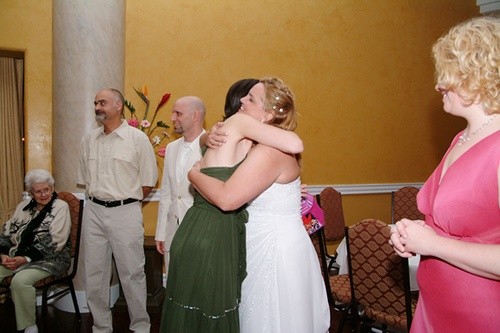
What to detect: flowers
<box><xmin>122</xmin><ymin>85</ymin><xmax>172</xmax><ymax>158</ymax></box>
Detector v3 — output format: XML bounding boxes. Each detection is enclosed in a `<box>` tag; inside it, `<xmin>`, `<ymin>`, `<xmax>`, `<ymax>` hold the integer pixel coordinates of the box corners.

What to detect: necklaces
<box><xmin>458</xmin><ymin>114</ymin><xmax>496</xmax><ymax>147</ymax></box>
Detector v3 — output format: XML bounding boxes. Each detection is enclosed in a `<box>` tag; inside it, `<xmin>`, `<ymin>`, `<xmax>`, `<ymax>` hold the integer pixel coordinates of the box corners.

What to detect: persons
<box><xmin>154</xmin><ymin>96</ymin><xmax>207</xmax><ymax>289</ymax></box>
<box><xmin>187</xmin><ymin>78</ymin><xmax>330</xmax><ymax>333</ymax></box>
<box><xmin>77</xmin><ymin>88</ymin><xmax>158</xmax><ymax>333</ymax></box>
<box><xmin>157</xmin><ymin>78</ymin><xmax>303</xmax><ymax>333</ymax></box>
<box><xmin>0</xmin><ymin>169</ymin><xmax>71</xmax><ymax>333</ymax></box>
<box><xmin>389</xmin><ymin>17</ymin><xmax>500</xmax><ymax>333</ymax></box>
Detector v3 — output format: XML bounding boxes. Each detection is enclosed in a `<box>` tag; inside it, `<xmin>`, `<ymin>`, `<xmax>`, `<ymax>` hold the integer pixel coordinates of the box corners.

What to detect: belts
<box><xmin>88</xmin><ymin>196</ymin><xmax>138</xmax><ymax>208</ymax></box>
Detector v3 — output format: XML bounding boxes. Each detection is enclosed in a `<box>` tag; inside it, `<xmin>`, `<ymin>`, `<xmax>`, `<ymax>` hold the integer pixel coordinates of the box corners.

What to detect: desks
<box><xmin>334</xmin><ymin>223</ymin><xmax>421</xmax><ymax>316</ymax></box>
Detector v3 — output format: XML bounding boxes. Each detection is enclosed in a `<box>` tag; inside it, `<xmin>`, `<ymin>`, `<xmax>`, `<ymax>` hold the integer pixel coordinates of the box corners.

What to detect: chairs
<box><xmin>0</xmin><ymin>191</ymin><xmax>85</xmax><ymax>333</ymax></box>
<box><xmin>315</xmin><ymin>186</ymin><xmax>353</xmax><ymax>333</ymax></box>
<box><xmin>345</xmin><ymin>218</ymin><xmax>419</xmax><ymax>333</ymax></box>
<box><xmin>391</xmin><ymin>187</ymin><xmax>425</xmax><ymax>224</ymax></box>
